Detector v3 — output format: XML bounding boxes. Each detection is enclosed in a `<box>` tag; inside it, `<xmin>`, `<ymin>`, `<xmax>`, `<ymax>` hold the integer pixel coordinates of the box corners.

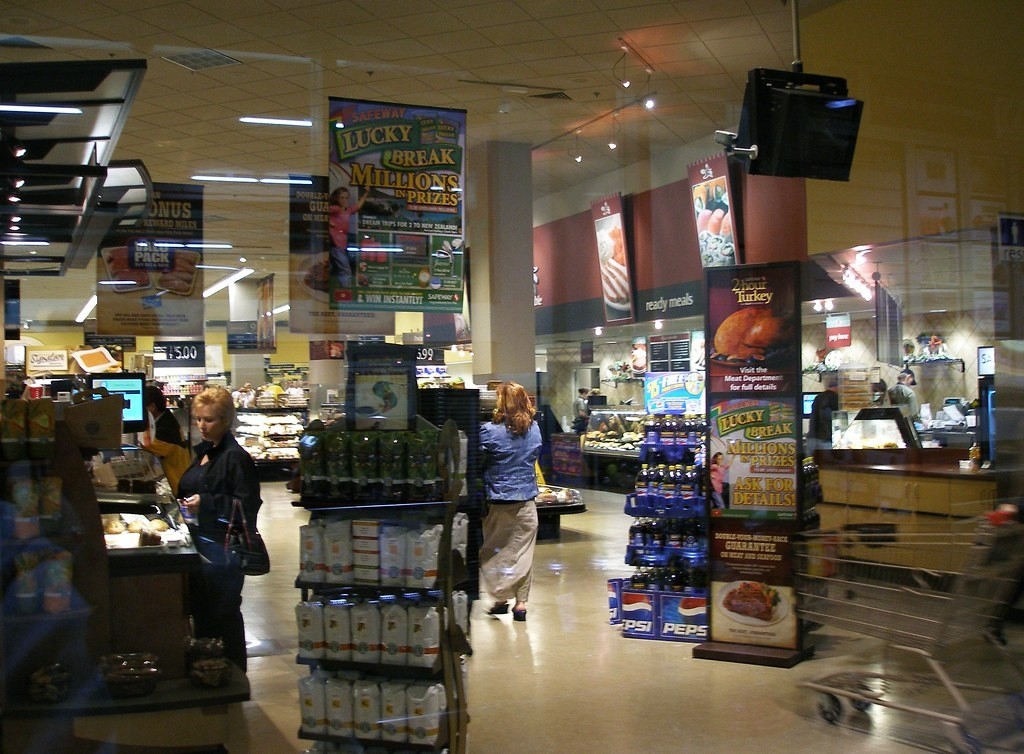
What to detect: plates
<box><xmin>296</xmin><ymin>252</ymin><xmax>330</xmax><ymax>303</ymax></box>
<box><xmin>717</xmin><ymin>580</ymin><xmax>787</xmax><ymax>626</ymax></box>
<box><xmin>101</xmin><ymin>246</ymin><xmax>153</xmax><ymax>292</ymax></box>
<box><xmin>154</xmin><ymin>250</ymin><xmax>201</xmax><ymax>295</ymax></box>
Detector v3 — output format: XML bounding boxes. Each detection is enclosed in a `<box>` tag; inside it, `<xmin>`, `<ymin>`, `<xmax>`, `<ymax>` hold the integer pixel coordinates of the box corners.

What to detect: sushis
<box><xmin>691</xmin><ymin>179</ymin><xmax>737</xmax><ymax>267</ymax></box>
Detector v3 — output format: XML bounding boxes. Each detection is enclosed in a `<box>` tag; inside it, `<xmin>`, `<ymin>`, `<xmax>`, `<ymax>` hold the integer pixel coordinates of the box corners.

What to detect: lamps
<box><xmin>708</xmin><ymin>52</ymin><xmax>873</xmax><ymax>192</ymax></box>
<box><xmin>262</xmin><ymin>303</ymin><xmax>290</xmax><ymax>318</ymax></box>
<box><xmin>74</xmin><ymin>293</ymin><xmax>99</xmax><ymax>325</ymax></box>
<box><xmin>201</xmin><ymin>268</ymin><xmax>255</xmax><ymax>299</ymax></box>
<box><xmin>811</xmin><ymin>265</ymin><xmax>875</xmax><ymax>314</ymax></box>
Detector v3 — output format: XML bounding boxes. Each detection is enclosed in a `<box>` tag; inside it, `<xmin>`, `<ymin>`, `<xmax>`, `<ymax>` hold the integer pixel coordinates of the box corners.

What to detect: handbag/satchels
<box><xmin>224</xmin><ymin>498</ymin><xmax>270</xmax><ymax>576</ymax></box>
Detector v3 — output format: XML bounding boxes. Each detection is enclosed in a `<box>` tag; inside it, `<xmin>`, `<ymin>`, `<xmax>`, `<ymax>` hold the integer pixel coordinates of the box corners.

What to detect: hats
<box><xmin>901</xmin><ymin>369</ymin><xmax>916</xmax><ymax>385</ymax></box>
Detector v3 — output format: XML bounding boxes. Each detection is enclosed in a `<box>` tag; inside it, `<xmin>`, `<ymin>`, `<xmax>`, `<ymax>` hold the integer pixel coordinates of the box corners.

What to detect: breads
<box><xmin>93</xmin><ymin>464</ymin><xmax>117</xmax><ymax>487</ymax></box>
<box><xmin>103</xmin><ymin>519</ymin><xmax>169</xmax><ymax>546</ymax></box>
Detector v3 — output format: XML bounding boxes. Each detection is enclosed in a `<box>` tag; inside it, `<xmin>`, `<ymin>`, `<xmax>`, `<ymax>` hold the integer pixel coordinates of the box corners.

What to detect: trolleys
<box><xmin>794</xmin><ymin>496</ymin><xmax>1024</xmax><ymax>754</ymax></box>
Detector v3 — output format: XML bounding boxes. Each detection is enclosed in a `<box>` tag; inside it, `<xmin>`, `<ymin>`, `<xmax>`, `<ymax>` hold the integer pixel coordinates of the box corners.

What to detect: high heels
<box><xmin>488</xmin><ymin>604</ymin><xmax>509</xmax><ymax>614</ymax></box>
<box><xmin>514</xmin><ymin>610</ymin><xmax>526</xmax><ymax>620</ymax></box>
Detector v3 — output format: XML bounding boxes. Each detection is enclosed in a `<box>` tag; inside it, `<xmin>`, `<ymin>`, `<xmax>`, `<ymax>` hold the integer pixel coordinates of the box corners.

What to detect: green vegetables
<box><xmin>765</xmin><ymin>587</ymin><xmax>781</xmax><ymax>606</ymax></box>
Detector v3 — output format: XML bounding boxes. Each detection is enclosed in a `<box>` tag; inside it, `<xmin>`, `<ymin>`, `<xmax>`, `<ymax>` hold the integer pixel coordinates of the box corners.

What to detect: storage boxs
<box><xmin>65</xmin><ymin>386</ymin><xmax>131</xmax><ymax>447</ymax></box>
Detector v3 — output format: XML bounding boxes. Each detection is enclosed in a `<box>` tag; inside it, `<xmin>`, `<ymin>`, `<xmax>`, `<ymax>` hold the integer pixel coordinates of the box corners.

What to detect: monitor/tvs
<box><xmin>762</xmin><ymin>88</ymin><xmax>864</xmax><ymax>182</ymax></box>
<box><xmin>86</xmin><ymin>372</ymin><xmax>148</xmax><ymax>432</ymax></box>
<box><xmin>802</xmin><ymin>392</ymin><xmax>822</xmax><ymax>419</ymax></box>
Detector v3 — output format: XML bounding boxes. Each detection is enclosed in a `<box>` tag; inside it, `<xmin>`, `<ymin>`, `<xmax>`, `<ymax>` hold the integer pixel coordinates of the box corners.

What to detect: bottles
<box><xmin>630</xmin><ymin>518</ymin><xmax>709</xmax><ymax>567</ymax></box>
<box><xmin>969</xmin><ymin>442</ymin><xmax>981</xmax><ymax>471</ymax></box>
<box><xmin>646</xmin><ymin>415</ymin><xmax>707</xmax><ymax>465</ymax></box>
<box><xmin>635</xmin><ymin>464</ymin><xmax>708</xmax><ymax>510</ymax></box>
<box><xmin>631</xmin><ymin>566</ymin><xmax>706</xmax><ymax>595</ymax></box>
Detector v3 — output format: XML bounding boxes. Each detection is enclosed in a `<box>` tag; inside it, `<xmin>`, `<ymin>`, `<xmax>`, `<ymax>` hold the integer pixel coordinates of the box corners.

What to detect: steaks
<box><xmin>721</xmin><ymin>582</ymin><xmax>772</xmax><ymax>621</ymax></box>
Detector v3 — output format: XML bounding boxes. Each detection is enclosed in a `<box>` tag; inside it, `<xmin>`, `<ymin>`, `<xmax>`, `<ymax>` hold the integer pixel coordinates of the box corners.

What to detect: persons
<box><xmin>479</xmin><ymin>380</ymin><xmax>542</xmax><ymax>621</ymax></box>
<box><xmin>144</xmin><ymin>385</ymin><xmax>191</xmax><ymax>496</ymax></box>
<box><xmin>175</xmin><ymin>387</ymin><xmax>263</xmax><ymax>674</ymax></box>
<box><xmin>887</xmin><ymin>369</ymin><xmax>916</xmax><ymax>416</ymax></box>
<box><xmin>328</xmin><ymin>186</ymin><xmax>370</xmax><ymax>287</ymax></box>
<box><xmin>710</xmin><ymin>452</ymin><xmax>736</xmax><ymax>509</ymax></box>
<box><xmin>174</xmin><ymin>399</ymin><xmax>189</xmax><ymax>426</ymax></box>
<box><xmin>573</xmin><ymin>388</ymin><xmax>588</xmax><ymax>418</ymax></box>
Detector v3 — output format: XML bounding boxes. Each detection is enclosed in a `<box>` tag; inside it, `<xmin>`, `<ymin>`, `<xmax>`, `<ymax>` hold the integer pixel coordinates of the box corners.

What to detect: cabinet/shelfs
<box><xmin>285</xmin><ymin>425</ymin><xmax>472</xmax><ymax>754</ymax></box>
<box><xmin>620</xmin><ymin>418</ymin><xmax>708</xmax><ymax>642</ymax></box>
<box><xmin>0</xmin><ymin>379</ymin><xmax>99</xmax><ymax>624</ymax></box>
<box><xmin>231</xmin><ymin>407</ymin><xmax>308</xmax><ymax>483</ymax></box>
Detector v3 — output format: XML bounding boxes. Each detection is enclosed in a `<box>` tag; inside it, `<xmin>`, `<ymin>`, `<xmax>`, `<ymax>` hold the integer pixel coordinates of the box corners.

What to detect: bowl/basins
<box><xmin>185</xmin><ymin>648</ymin><xmax>223</xmax><ymax>667</ymax></box>
<box><xmin>192</xmin><ymin>660</ymin><xmax>232</xmax><ymax>690</ymax></box>
<box><xmin>99</xmin><ymin>676</ymin><xmax>158</xmax><ymax>700</ymax></box>
